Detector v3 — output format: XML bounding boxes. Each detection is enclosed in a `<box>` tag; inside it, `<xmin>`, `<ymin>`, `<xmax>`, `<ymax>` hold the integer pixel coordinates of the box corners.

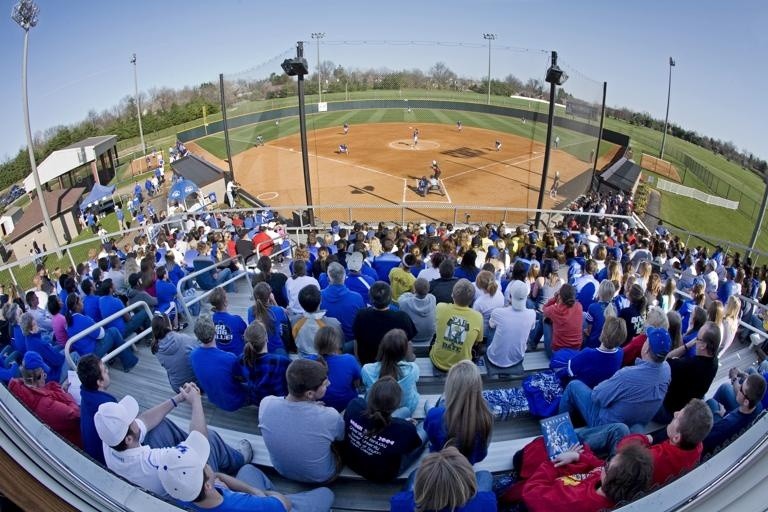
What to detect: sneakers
<box><xmin>173</xmin><ymin>323</ymin><xmax>187</xmax><ymax>330</ymax></box>
<box><xmin>235</xmin><ymin>439</ymin><xmax>253</xmax><ymax>464</ymax></box>
<box><xmin>424</xmin><ymin>400</ymin><xmax>431</xmax><ymax>413</ymax></box>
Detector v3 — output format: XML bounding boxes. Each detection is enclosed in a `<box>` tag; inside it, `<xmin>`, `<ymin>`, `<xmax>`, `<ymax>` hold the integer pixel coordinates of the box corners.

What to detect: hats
<box><xmin>544</xmin><ymin>258</ymin><xmax>558</xmax><ymax>271</ymax></box>
<box><xmin>94</xmin><ymin>394</ymin><xmax>139</xmax><ymax>447</ymax></box>
<box><xmin>23</xmin><ymin>351</ymin><xmax>52</xmax><ymax>373</ymax></box>
<box><xmin>488</xmin><ymin>246</ymin><xmax>497</xmax><ymax>256</ymax></box>
<box><xmin>607</xmin><ymin>247</ymin><xmax>622</xmax><ymax>257</ymax></box>
<box><xmin>347</xmin><ymin>251</ymin><xmax>363</xmax><ymax>272</ymax></box>
<box><xmin>159</xmin><ymin>430</ymin><xmax>211</xmax><ymax>502</ymax></box>
<box><xmin>645</xmin><ymin>327</ymin><xmax>672</xmax><ymax>359</ymax></box>
<box><xmin>129</xmin><ymin>272</ymin><xmax>142</xmax><ymax>286</ymax></box>
<box><xmin>510</xmin><ymin>280</ymin><xmax>529</xmax><ymax>310</ymax></box>
<box><xmin>725</xmin><ymin>268</ymin><xmax>735</xmax><ymax>277</ymax></box>
<box><xmin>527</xmin><ymin>233</ymin><xmax>536</xmax><ymax>240</ymax></box>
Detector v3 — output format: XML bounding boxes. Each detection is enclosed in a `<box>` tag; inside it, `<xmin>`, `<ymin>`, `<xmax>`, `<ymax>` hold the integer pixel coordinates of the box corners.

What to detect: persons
<box><xmin>0</xmin><ymin>117</ymin><xmax>767</xmax><ymax>512</ymax></box>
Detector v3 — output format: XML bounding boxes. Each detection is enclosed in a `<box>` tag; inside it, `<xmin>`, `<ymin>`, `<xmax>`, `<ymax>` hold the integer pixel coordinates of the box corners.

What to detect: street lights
<box><xmin>7</xmin><ymin>0</ymin><xmax>67</xmax><ymax>262</ymax></box>
<box><xmin>657</xmin><ymin>54</ymin><xmax>676</xmax><ymax>157</ymax></box>
<box><xmin>530</xmin><ymin>47</ymin><xmax>571</xmax><ymax>229</ymax></box>
<box><xmin>279</xmin><ymin>37</ymin><xmax>315</xmax><ymax>229</ymax></box>
<box><xmin>129</xmin><ymin>50</ymin><xmax>148</xmax><ymax>156</ymax></box>
<box><xmin>310</xmin><ymin>29</ymin><xmax>326</xmax><ymax>103</ymax></box>
<box><xmin>480</xmin><ymin>30</ymin><xmax>498</xmax><ymax>105</ymax></box>
